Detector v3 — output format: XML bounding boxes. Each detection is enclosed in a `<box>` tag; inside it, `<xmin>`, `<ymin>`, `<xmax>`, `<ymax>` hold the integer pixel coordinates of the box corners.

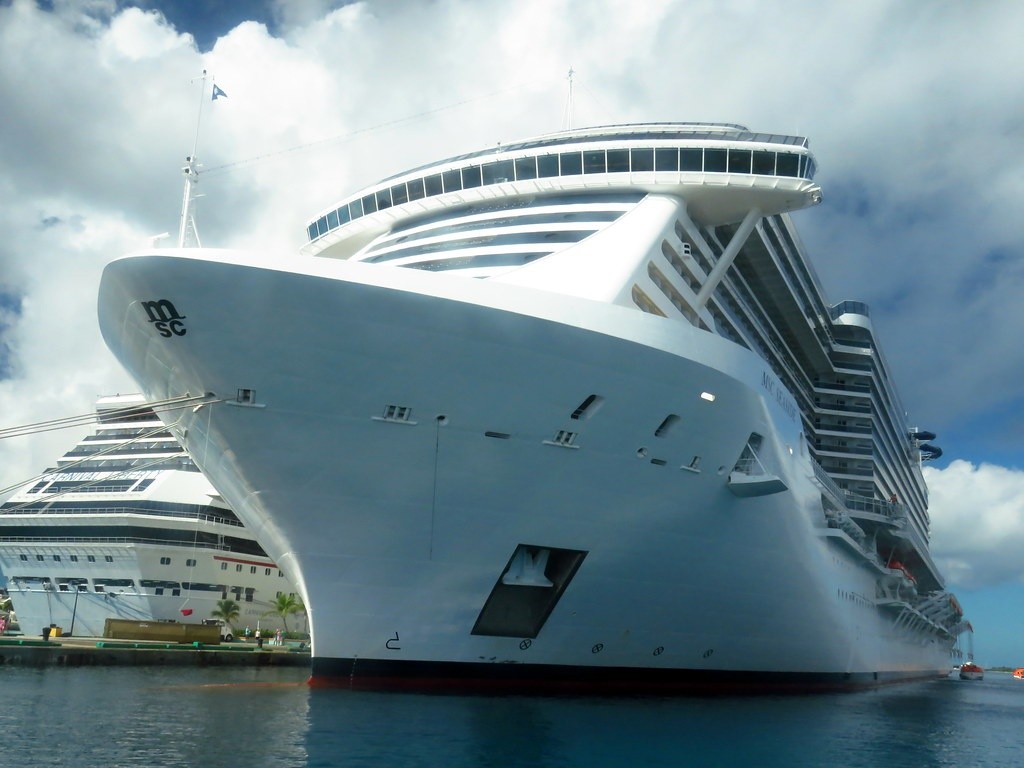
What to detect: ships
<box><xmin>0</xmin><ymin>157</ymin><xmax>314</xmax><ymax>642</ymax></box>
<box><xmin>95</xmin><ymin>60</ymin><xmax>974</xmax><ymax>697</ymax></box>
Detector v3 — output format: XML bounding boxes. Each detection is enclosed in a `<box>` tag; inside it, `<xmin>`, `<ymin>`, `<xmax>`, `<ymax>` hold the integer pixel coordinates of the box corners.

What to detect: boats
<box><xmin>958</xmin><ymin>664</ymin><xmax>984</xmax><ymax>681</ymax></box>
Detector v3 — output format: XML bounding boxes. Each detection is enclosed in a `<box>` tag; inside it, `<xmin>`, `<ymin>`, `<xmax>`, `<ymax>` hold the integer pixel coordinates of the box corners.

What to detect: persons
<box><xmin>888</xmin><ymin>493</ymin><xmax>899</xmax><ymax>505</ymax></box>
<box><xmin>245</xmin><ymin>625</ymin><xmax>252</xmax><ymax>641</ymax></box>
<box><xmin>273</xmin><ymin>628</ymin><xmax>286</xmax><ymax>646</ymax></box>
<box><xmin>254</xmin><ymin>628</ymin><xmax>261</xmax><ymax>642</ymax></box>
